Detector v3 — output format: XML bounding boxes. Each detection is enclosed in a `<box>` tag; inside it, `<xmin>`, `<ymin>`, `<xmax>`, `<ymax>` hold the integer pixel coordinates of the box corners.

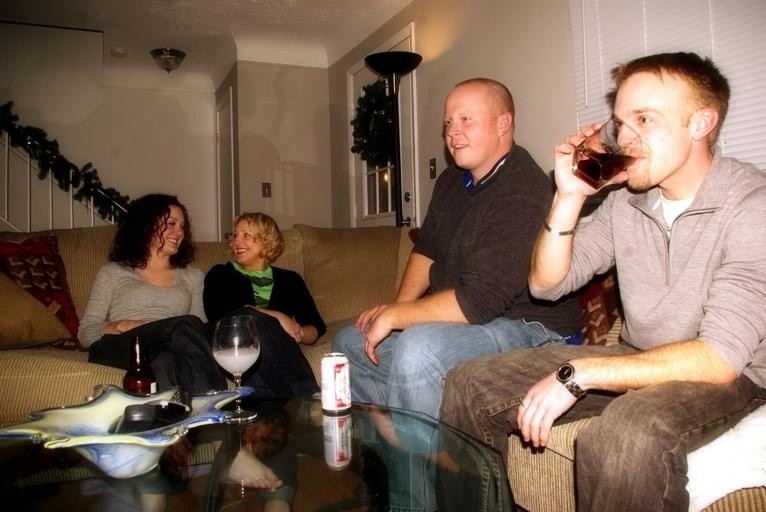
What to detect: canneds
<box><xmin>323</xmin><ymin>414</ymin><xmax>352</xmax><ymax>470</ymax></box>
<box><xmin>320</xmin><ymin>353</ymin><xmax>352</xmax><ymax>413</ymax></box>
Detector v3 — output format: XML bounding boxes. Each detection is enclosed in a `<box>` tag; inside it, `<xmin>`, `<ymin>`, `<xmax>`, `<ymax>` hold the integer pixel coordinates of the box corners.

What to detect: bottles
<box><xmin>122</xmin><ymin>334</ymin><xmax>160</xmax><ymax>422</ymax></box>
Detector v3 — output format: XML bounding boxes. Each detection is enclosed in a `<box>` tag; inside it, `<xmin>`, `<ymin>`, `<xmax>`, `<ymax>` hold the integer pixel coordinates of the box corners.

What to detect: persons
<box><xmin>430</xmin><ymin>49</ymin><xmax>766</xmax><ymax>510</ymax></box>
<box><xmin>77</xmin><ymin>193</ymin><xmax>286</xmax><ymax>511</ymax></box>
<box><xmin>200</xmin><ymin>212</ymin><xmax>328</xmax><ymax>511</ymax></box>
<box><xmin>332</xmin><ymin>77</ymin><xmax>584</xmax><ymax>509</ymax></box>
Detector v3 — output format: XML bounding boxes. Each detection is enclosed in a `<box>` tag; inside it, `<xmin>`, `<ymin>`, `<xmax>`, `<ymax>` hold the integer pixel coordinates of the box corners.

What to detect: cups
<box><xmin>572</xmin><ymin>121</ymin><xmax>643</xmax><ymax>187</ymax></box>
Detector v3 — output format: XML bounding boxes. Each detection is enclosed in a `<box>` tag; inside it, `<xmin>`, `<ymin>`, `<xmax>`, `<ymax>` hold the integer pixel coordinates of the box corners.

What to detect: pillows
<box><xmin>0</xmin><ymin>228</ymin><xmax>89</xmax><ymax>351</ymax></box>
<box><xmin>293</xmin><ymin>222</ymin><xmax>400</xmax><ymax>328</ymax></box>
<box><xmin>188</xmin><ymin>229</ymin><xmax>304</xmax><ymax>283</ymax></box>
<box><xmin>0</xmin><ymin>270</ymin><xmax>73</xmax><ymax>352</ymax></box>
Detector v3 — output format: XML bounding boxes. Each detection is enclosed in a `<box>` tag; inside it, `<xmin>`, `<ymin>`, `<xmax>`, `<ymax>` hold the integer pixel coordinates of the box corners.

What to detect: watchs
<box><xmin>558</xmin><ymin>359</ymin><xmax>587</xmax><ymax>402</ymax></box>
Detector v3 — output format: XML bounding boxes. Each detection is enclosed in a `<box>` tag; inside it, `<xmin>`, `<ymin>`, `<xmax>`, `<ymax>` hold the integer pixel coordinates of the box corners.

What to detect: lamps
<box><xmin>364</xmin><ymin>51</ymin><xmax>423</xmax><ymax>225</ymax></box>
<box><xmin>150</xmin><ymin>46</ymin><xmax>186</xmax><ymax>72</ymax></box>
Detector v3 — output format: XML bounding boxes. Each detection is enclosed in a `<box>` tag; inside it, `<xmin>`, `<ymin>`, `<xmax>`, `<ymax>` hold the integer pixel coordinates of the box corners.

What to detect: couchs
<box><xmin>1</xmin><ymin>223</ymin><xmax>766</xmax><ymax>511</ymax></box>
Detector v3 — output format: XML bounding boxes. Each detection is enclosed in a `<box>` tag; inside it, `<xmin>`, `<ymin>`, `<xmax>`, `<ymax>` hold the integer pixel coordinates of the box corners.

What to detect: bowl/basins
<box><xmin>0</xmin><ymin>382</ymin><xmax>257</xmax><ymax>479</ymax></box>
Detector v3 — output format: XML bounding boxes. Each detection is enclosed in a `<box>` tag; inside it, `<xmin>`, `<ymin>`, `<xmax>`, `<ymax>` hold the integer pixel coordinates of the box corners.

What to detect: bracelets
<box><xmin>542</xmin><ymin>218</ymin><xmax>575</xmax><ymax>239</ymax></box>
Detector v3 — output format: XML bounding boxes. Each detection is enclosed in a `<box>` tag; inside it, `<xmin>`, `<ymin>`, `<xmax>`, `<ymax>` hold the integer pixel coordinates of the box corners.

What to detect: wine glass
<box><xmin>213</xmin><ymin>315</ymin><xmax>262</xmax><ymax>420</ymax></box>
